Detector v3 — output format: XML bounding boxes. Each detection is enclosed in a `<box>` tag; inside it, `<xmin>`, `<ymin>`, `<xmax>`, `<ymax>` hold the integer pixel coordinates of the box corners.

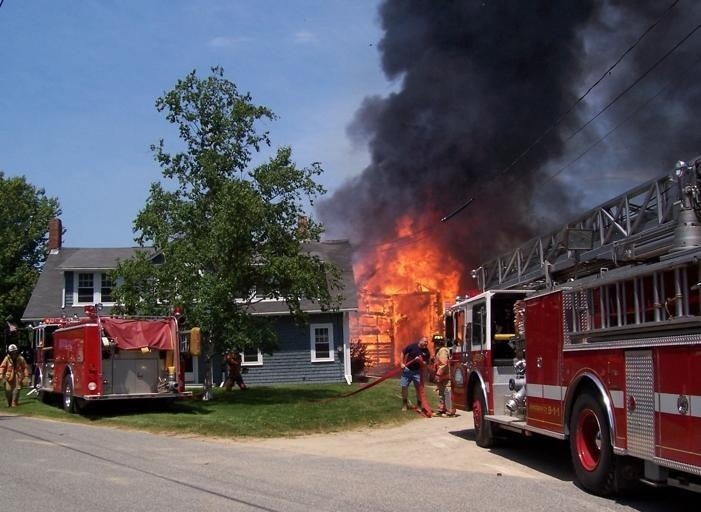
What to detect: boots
<box><xmin>415</xmin><ymin>400</ymin><xmax>421</xmax><ymax>411</ymax></box>
<box><xmin>7</xmin><ymin>400</ymin><xmax>16</xmax><ymax>407</ymax></box>
<box><xmin>240</xmin><ymin>383</ymin><xmax>246</xmax><ymax>390</ymax></box>
<box><xmin>402</xmin><ymin>398</ymin><xmax>408</xmax><ymax>412</ymax></box>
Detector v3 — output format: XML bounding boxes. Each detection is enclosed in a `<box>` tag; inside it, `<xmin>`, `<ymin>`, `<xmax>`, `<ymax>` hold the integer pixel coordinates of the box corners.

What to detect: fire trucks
<box><xmin>444</xmin><ymin>159</ymin><xmax>701</xmax><ymax>496</ymax></box>
<box><xmin>31</xmin><ymin>305</ymin><xmax>193</xmax><ymax>411</ymax></box>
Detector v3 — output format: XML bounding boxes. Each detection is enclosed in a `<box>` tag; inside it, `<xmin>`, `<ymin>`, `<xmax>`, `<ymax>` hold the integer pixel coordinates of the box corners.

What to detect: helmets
<box><xmin>431</xmin><ymin>334</ymin><xmax>445</xmax><ymax>349</ymax></box>
<box><xmin>8</xmin><ymin>344</ymin><xmax>18</xmax><ymax>352</ymax></box>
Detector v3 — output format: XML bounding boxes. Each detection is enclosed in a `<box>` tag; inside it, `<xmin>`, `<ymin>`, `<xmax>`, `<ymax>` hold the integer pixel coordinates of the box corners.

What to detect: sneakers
<box><xmin>433</xmin><ymin>412</ymin><xmax>456</xmax><ymax>418</ymax></box>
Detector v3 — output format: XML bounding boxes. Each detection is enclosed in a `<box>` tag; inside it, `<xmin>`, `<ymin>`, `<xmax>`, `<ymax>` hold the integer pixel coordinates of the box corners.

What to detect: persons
<box><xmin>431</xmin><ymin>336</ymin><xmax>456</xmax><ymax>418</ymax></box>
<box><xmin>222</xmin><ymin>348</ymin><xmax>247</xmax><ymax>391</ymax></box>
<box><xmin>401</xmin><ymin>335</ymin><xmax>432</xmax><ymax>412</ymax></box>
<box><xmin>0</xmin><ymin>344</ymin><xmax>30</xmax><ymax>408</ymax></box>
